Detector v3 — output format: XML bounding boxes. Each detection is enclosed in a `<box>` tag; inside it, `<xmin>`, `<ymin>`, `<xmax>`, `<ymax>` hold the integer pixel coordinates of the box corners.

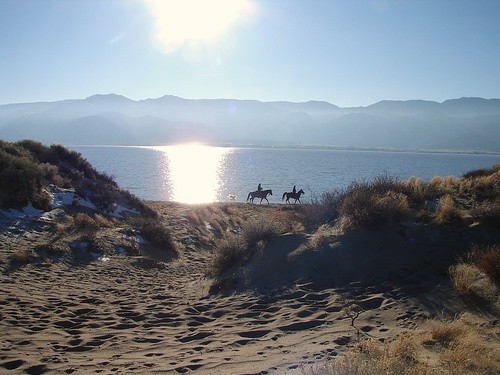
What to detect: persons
<box><xmin>293</xmin><ymin>185</ymin><xmax>296</xmax><ymax>193</ymax></box>
<box><xmin>258</xmin><ymin>183</ymin><xmax>263</xmax><ymax>191</ymax></box>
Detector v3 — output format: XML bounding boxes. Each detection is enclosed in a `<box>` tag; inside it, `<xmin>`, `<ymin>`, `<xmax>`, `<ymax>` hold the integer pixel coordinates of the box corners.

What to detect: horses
<box><xmin>282</xmin><ymin>188</ymin><xmax>305</xmax><ymax>205</ymax></box>
<box><xmin>246</xmin><ymin>189</ymin><xmax>272</xmax><ymax>206</ymax></box>
